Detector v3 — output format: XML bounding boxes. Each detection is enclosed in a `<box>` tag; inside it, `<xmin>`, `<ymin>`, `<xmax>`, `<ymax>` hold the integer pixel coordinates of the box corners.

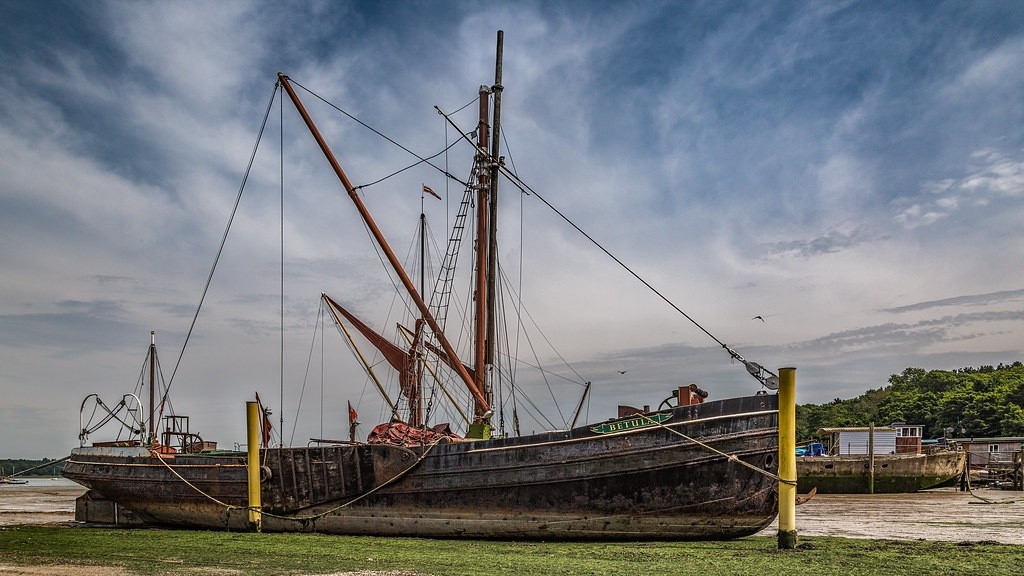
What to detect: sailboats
<box><xmin>59</xmin><ymin>71</ymin><xmax>786</xmax><ymax>541</ymax></box>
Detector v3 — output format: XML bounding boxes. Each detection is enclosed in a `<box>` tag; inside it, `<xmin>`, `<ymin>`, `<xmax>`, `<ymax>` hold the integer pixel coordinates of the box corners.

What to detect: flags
<box><xmin>349</xmin><ymin>405</ymin><xmax>358</xmax><ymax>426</ymax></box>
<box><xmin>262</xmin><ymin>412</ymin><xmax>273</xmax><ymax>448</ymax></box>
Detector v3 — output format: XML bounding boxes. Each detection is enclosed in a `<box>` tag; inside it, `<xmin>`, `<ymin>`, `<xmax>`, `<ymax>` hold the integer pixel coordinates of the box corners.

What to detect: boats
<box><xmin>792</xmin><ymin>451</ymin><xmax>968</xmax><ymax>494</ymax></box>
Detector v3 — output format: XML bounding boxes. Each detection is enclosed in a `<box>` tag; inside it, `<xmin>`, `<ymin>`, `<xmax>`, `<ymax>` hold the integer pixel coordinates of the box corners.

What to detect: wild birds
<box><xmin>480</xmin><ymin>409</ymin><xmax>496</xmax><ymax>424</ymax></box>
<box><xmin>751</xmin><ymin>316</ymin><xmax>764</xmax><ymax>322</ymax></box>
<box><xmin>618</xmin><ymin>370</ymin><xmax>627</xmax><ymax>375</ymax></box>
